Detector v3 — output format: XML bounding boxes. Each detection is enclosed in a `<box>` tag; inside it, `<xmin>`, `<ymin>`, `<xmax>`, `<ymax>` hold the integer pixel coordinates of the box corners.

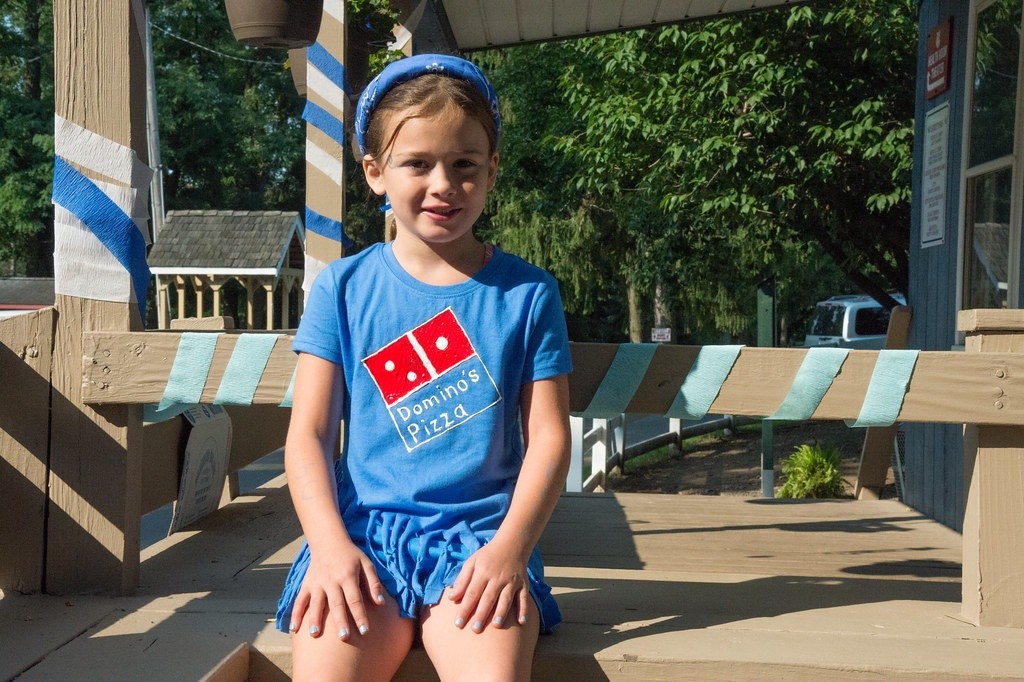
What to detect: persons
<box><xmin>275</xmin><ymin>54</ymin><xmax>575</xmax><ymax>682</ymax></box>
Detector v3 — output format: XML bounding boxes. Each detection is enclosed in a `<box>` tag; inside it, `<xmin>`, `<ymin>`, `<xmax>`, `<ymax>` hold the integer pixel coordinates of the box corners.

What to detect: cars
<box><xmin>806</xmin><ymin>288</ymin><xmax>907</xmax><ymax>349</ymax></box>
